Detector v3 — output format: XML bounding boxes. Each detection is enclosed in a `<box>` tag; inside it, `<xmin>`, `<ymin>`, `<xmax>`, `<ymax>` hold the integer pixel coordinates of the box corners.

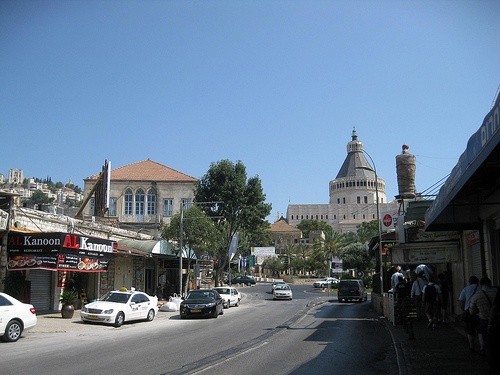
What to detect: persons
<box><xmin>458</xmin><ymin>276</ymin><xmax>484</xmax><ymax>351</ymax></box>
<box><xmin>391</xmin><ymin>263</ymin><xmax>449</xmax><ymax>326</ymax></box>
<box><xmin>470</xmin><ymin>276</ymin><xmax>500</xmax><ymax>353</ymax></box>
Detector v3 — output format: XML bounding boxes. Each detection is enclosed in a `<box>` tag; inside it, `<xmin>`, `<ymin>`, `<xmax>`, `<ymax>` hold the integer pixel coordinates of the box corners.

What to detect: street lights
<box><xmin>355</xmin><ymin>165</ymin><xmax>384</xmax><ymax>295</ymax></box>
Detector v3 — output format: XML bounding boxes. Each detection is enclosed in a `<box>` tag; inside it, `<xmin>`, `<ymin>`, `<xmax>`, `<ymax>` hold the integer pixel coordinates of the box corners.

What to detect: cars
<box><xmin>225</xmin><ymin>275</ymin><xmax>256</xmax><ymax>286</ymax></box>
<box><xmin>80</xmin><ymin>287</ymin><xmax>159</xmax><ymax>327</ymax></box>
<box><xmin>214</xmin><ymin>287</ymin><xmax>241</xmax><ymax>308</ymax></box>
<box><xmin>0</xmin><ymin>292</ymin><xmax>37</xmax><ymax>341</ymax></box>
<box><xmin>272</xmin><ymin>282</ymin><xmax>285</xmax><ymax>293</ymax></box>
<box><xmin>313</xmin><ymin>278</ymin><xmax>340</xmax><ymax>288</ymax></box>
<box><xmin>180</xmin><ymin>290</ymin><xmax>223</xmax><ymax>318</ymax></box>
<box><xmin>273</xmin><ymin>284</ymin><xmax>292</xmax><ymax>300</ymax></box>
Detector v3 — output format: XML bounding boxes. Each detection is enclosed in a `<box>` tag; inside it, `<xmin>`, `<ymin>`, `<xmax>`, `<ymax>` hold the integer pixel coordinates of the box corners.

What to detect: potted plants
<box><xmin>59</xmin><ymin>289</ymin><xmax>77</xmax><ymax>319</ymax></box>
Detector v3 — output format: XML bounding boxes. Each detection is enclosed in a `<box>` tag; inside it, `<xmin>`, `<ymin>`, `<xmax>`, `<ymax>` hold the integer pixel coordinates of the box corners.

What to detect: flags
<box><xmin>228</xmin><ymin>231</ymin><xmax>239</xmax><ymax>261</ymax></box>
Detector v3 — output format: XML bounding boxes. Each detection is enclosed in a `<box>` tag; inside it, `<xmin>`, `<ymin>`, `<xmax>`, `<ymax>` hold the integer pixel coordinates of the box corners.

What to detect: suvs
<box><xmin>338</xmin><ymin>279</ymin><xmax>368</xmax><ymax>302</ymax></box>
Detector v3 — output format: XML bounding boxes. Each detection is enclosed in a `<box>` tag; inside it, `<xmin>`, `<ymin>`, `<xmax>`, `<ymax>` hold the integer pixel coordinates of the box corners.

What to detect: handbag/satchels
<box><xmin>462</xmin><ymin>307</ymin><xmax>470</xmax><ymax>322</ymax></box>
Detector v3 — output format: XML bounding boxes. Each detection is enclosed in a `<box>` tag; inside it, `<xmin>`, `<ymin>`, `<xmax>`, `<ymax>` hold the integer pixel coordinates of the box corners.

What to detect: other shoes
<box><xmin>438</xmin><ymin>319</ymin><xmax>447</xmax><ymax>325</ymax></box>
<box><xmin>427</xmin><ymin>320</ymin><xmax>436</xmax><ymax>330</ymax></box>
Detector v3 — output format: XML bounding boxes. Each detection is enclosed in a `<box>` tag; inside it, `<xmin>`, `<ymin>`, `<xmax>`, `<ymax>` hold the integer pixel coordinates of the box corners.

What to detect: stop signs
<box><xmin>382</xmin><ymin>214</ymin><xmax>392</xmax><ymax>227</ymax></box>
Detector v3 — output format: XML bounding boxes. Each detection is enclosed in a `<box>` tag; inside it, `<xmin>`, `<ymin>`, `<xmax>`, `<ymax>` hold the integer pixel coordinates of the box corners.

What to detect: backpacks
<box><xmin>424</xmin><ymin>283</ymin><xmax>437</xmax><ymax>304</ymax></box>
